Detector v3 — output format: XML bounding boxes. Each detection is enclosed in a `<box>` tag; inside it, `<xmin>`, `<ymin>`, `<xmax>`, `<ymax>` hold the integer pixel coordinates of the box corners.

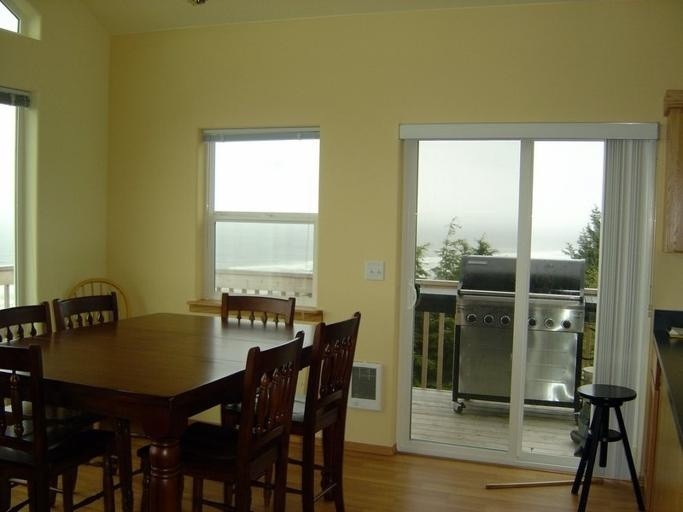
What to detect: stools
<box><xmin>571</xmin><ymin>384</ymin><xmax>646</xmax><ymax>512</ymax></box>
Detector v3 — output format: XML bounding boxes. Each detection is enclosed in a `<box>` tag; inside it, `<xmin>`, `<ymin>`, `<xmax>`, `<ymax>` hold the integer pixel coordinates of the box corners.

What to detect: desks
<box><xmin>1</xmin><ymin>312</ymin><xmax>306</xmax><ymax>509</ymax></box>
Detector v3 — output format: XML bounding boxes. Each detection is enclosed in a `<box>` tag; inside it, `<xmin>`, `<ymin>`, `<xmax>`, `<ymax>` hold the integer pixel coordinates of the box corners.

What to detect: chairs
<box><xmin>258</xmin><ymin>309</ymin><xmax>361</xmax><ymax>511</ymax></box>
<box><xmin>1</xmin><ymin>301</ymin><xmax>118</xmax><ymax>510</ymax></box>
<box><xmin>171</xmin><ymin>292</ymin><xmax>297</xmax><ymax>491</ymax></box>
<box><xmin>136</xmin><ymin>327</ymin><xmax>305</xmax><ymax>512</ymax></box>
<box><xmin>51</xmin><ymin>289</ymin><xmax>177</xmax><ymax>511</ymax></box>
